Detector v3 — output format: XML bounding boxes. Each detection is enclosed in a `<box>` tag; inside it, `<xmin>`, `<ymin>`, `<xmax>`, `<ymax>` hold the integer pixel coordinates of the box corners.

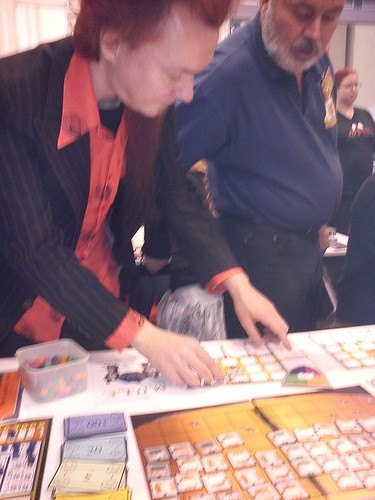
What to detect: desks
<box><xmin>0</xmin><ymin>321</ymin><xmax>375</xmax><ymax>500</ymax></box>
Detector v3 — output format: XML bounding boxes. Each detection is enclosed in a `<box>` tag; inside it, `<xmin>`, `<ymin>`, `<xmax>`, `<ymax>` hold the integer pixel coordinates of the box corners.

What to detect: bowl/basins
<box><xmin>15</xmin><ymin>337</ymin><xmax>89</xmax><ymax>402</ymax></box>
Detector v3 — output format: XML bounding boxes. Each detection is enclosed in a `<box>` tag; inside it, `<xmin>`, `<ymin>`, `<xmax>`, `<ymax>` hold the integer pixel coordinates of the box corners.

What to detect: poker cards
<box><xmin>47</xmin><ymin>412</ymin><xmax>132</xmax><ymax>500</ymax></box>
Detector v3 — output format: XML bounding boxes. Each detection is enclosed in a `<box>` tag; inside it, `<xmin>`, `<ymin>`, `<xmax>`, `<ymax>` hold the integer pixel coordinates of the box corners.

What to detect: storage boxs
<box><xmin>15</xmin><ymin>338</ymin><xmax>90</xmax><ymax>404</ymax></box>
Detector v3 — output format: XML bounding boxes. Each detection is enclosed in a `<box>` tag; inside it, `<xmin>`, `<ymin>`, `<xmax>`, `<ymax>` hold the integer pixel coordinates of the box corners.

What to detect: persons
<box><xmin>0</xmin><ymin>0</ymin><xmax>291</xmax><ymax>386</ymax></box>
<box><xmin>142</xmin><ymin>0</ymin><xmax>343</xmax><ymax>339</ymax></box>
<box><xmin>333</xmin><ymin>69</ymin><xmax>375</xmax><ymax>236</ymax></box>
<box><xmin>337</xmin><ymin>173</ymin><xmax>375</xmax><ymax>325</ymax></box>
<box><xmin>142</xmin><ymin>161</ymin><xmax>227</xmax><ymax>341</ymax></box>
